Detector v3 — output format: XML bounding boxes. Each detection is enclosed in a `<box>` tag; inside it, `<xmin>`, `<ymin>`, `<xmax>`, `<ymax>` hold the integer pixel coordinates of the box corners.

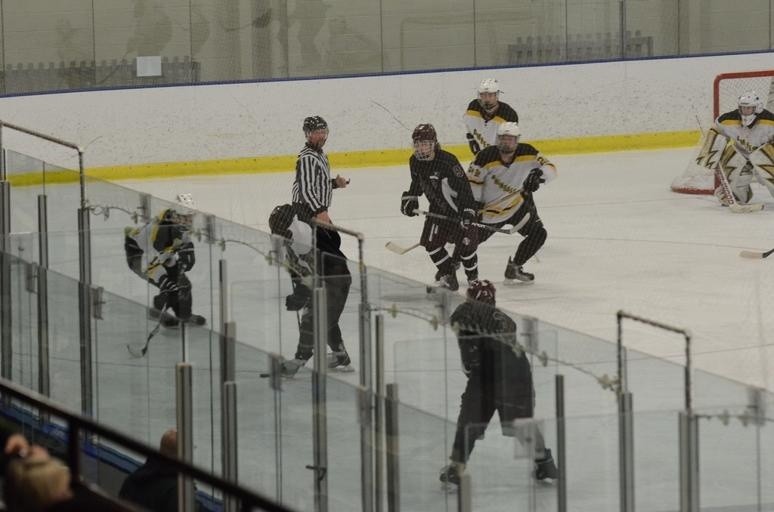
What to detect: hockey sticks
<box><xmin>385</xmin><ymin>188</ymin><xmax>525</xmax><ymax>255</ymax></box>
<box><xmin>740</xmin><ymin>249</ymin><xmax>774</xmax><ymax>259</ymax></box>
<box><xmin>691</xmin><ymin>104</ymin><xmax>764</xmax><ymax>212</ymax></box>
<box><xmin>412</xmin><ymin>209</ymin><xmax>530</xmax><ymax>234</ymax></box>
<box><xmin>127</xmin><ymin>265</ymin><xmax>187</xmax><ymax>358</ymax></box>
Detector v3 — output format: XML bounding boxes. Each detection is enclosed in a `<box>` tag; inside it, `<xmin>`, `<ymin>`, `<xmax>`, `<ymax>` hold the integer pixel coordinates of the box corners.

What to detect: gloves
<box><xmin>177</xmin><ymin>242</ymin><xmax>196</xmax><ymax>272</ymax></box>
<box><xmin>524</xmin><ymin>168</ymin><xmax>545</xmax><ymax>192</ymax></box>
<box><xmin>158</xmin><ymin>274</ymin><xmax>183</xmax><ymax>300</ymax></box>
<box><xmin>400</xmin><ymin>190</ymin><xmax>421</xmax><ymax>217</ymax></box>
<box><xmin>458</xmin><ymin>211</ymin><xmax>477</xmax><ymax>232</ymax></box>
<box><xmin>286</xmin><ymin>285</ymin><xmax>308</xmax><ymax>312</ymax></box>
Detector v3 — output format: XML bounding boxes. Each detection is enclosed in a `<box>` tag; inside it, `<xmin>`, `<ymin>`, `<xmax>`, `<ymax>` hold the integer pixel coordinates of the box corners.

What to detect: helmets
<box><xmin>268</xmin><ymin>203</ymin><xmax>296</xmax><ymax>243</ymax></box>
<box><xmin>303</xmin><ymin>116</ymin><xmax>329</xmax><ymax>148</ymax></box>
<box><xmin>411</xmin><ymin>123</ymin><xmax>438</xmax><ymax>162</ymax></box>
<box><xmin>737</xmin><ymin>90</ymin><xmax>765</xmax><ymax>128</ymax></box>
<box><xmin>476</xmin><ymin>77</ymin><xmax>501</xmax><ymax>110</ymax></box>
<box><xmin>465</xmin><ymin>279</ymin><xmax>497</xmax><ymax>307</ymax></box>
<box><xmin>169</xmin><ymin>192</ymin><xmax>196</xmax><ymax>231</ymax></box>
<box><xmin>496</xmin><ymin>122</ymin><xmax>521</xmax><ymax>153</ymax></box>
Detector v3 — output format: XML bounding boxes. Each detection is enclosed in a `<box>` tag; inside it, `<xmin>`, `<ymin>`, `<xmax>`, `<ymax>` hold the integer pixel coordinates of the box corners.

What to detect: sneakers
<box><xmin>530</xmin><ymin>448</ymin><xmax>558</xmax><ymax>480</ymax></box>
<box><xmin>326</xmin><ymin>350</ymin><xmax>350</xmax><ymax>369</ymax></box>
<box><xmin>180</xmin><ymin>314</ymin><xmax>207</xmax><ymax>326</ymax></box>
<box><xmin>439</xmin><ymin>455</ymin><xmax>467</xmax><ymax>486</ymax></box>
<box><xmin>426</xmin><ymin>261</ymin><xmax>461</xmax><ymax>294</ymax></box>
<box><xmin>277</xmin><ymin>358</ymin><xmax>303</xmax><ymax>377</ymax></box>
<box><xmin>151</xmin><ymin>309</ymin><xmax>179</xmax><ymax>326</ymax></box>
<box><xmin>506</xmin><ymin>256</ymin><xmax>535</xmax><ymax>282</ymax></box>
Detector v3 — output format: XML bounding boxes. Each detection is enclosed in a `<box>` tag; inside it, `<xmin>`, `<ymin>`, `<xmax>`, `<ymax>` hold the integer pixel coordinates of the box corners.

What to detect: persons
<box><xmin>124</xmin><ymin>191</ymin><xmax>206</xmax><ymax>330</ymax></box>
<box><xmin>292</xmin><ymin>115</ymin><xmax>348</xmax><ymax>248</ymax></box>
<box><xmin>450</xmin><ymin>121</ymin><xmax>566</xmax><ymax>282</ymax></box>
<box><xmin>438</xmin><ymin>279</ymin><xmax>557</xmax><ymax>484</ymax></box>
<box><xmin>460</xmin><ymin>77</ymin><xmax>518</xmax><ymax>153</ymax></box>
<box><xmin>269</xmin><ymin>203</ymin><xmax>352</xmax><ymax>380</ymax></box>
<box><xmin>400</xmin><ymin>123</ymin><xmax>480</xmax><ymax>296</ymax></box>
<box><xmin>713</xmin><ymin>89</ymin><xmax>774</xmax><ymax>207</ymax></box>
<box><xmin>117</xmin><ymin>425</ymin><xmax>210</xmax><ymax>511</ymax></box>
<box><xmin>0</xmin><ymin>432</ymin><xmax>79</xmax><ymax>512</ymax></box>
